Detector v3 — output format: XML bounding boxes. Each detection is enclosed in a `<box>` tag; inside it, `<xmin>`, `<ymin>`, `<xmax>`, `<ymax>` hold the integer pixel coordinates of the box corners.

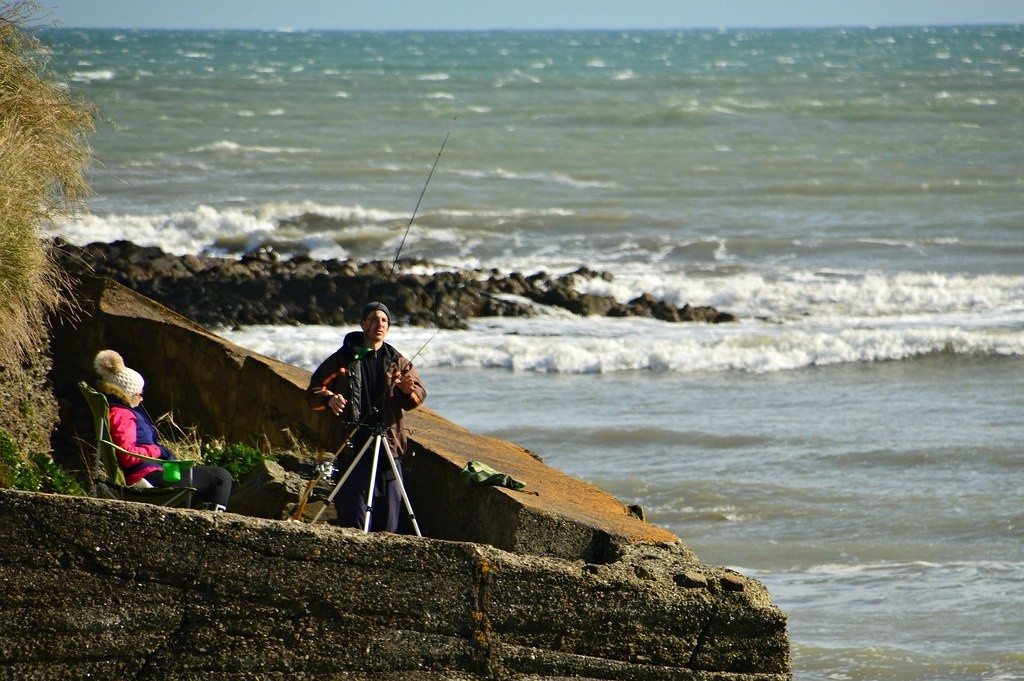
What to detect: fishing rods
<box><xmin>284</xmin><ymin>294</ymin><xmax>484</xmax><ymax>521</ymax></box>
<box><xmin>341</xmin><ymin>112</ymin><xmax>460</xmax><ymax>394</ymax></box>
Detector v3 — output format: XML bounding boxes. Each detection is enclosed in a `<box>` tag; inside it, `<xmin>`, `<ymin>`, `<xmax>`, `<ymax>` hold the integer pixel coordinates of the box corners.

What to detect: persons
<box><xmin>94</xmin><ymin>349</ymin><xmax>232</xmax><ymax>513</ymax></box>
<box><xmin>306</xmin><ymin>301</ymin><xmax>428</xmax><ymax>533</ymax></box>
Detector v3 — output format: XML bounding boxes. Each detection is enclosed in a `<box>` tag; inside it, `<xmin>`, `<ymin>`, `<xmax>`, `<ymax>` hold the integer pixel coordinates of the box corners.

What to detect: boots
<box><xmin>202</xmin><ymin>502</ymin><xmax>226</xmax><ymax>513</ymax></box>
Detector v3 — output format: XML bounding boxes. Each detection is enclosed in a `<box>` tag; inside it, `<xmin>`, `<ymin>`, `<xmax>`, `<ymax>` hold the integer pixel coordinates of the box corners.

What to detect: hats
<box><xmin>94</xmin><ymin>350</ymin><xmax>145</xmax><ymax>399</ymax></box>
<box><xmin>361</xmin><ymin>302</ymin><xmax>391</xmax><ymax>327</ymax></box>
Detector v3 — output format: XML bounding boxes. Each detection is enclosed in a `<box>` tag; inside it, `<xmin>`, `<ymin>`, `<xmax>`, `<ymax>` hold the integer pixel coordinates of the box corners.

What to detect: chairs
<box><xmin>78</xmin><ymin>381</ymin><xmax>198</xmax><ymax>509</ymax></box>
